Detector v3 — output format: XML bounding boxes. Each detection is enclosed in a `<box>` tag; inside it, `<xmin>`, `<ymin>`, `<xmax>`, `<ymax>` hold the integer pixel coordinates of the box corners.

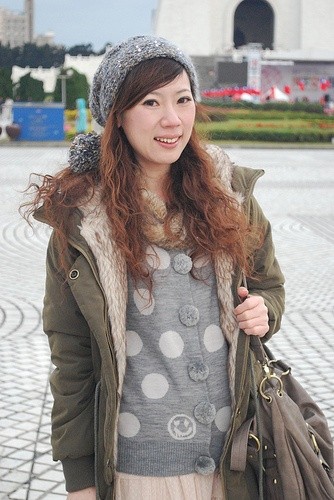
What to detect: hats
<box><xmin>68</xmin><ymin>33</ymin><xmax>201</xmax><ymax>175</ymax></box>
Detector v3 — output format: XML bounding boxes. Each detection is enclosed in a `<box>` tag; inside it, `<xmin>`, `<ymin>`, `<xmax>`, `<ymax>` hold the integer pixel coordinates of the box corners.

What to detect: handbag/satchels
<box><xmin>230</xmin><ymin>359</ymin><xmax>334</xmax><ymax>500</ymax></box>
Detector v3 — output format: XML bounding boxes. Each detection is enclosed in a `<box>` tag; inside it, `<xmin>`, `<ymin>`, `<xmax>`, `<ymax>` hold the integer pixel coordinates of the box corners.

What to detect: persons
<box><xmin>20</xmin><ymin>33</ymin><xmax>286</xmax><ymax>498</ymax></box>
